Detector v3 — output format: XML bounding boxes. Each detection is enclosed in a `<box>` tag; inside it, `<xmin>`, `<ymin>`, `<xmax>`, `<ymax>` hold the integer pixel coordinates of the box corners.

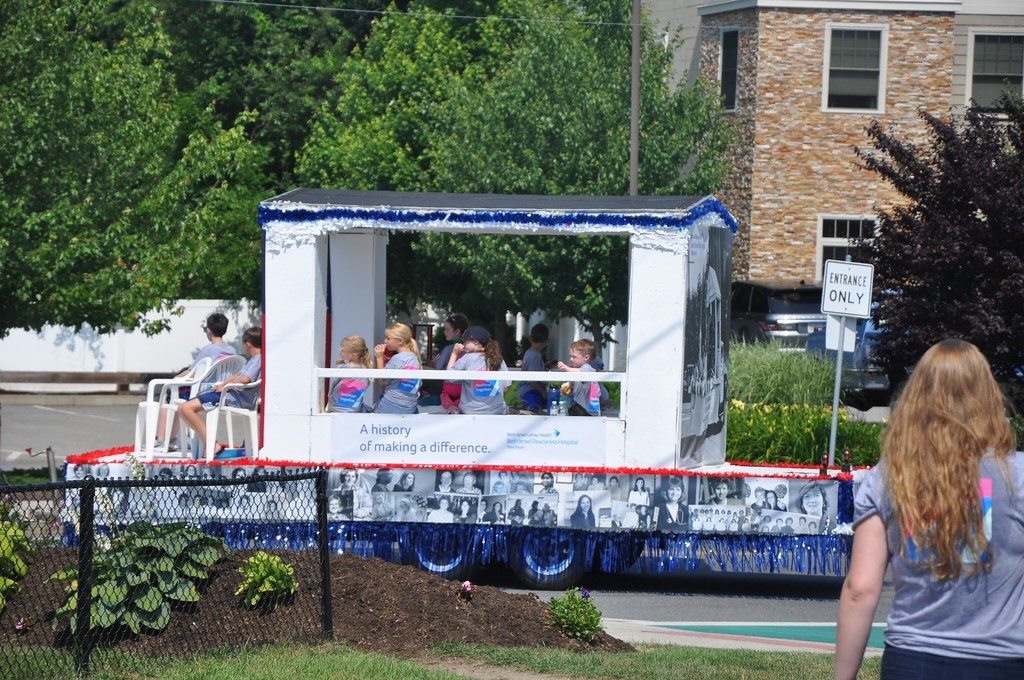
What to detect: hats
<box><xmin>591</xmin><ymin>356</ymin><xmax>604</xmax><ymax>367</ymax></box>
<box><xmin>462</xmin><ymin>325</ymin><xmax>492</xmax><ymax>345</ymax></box>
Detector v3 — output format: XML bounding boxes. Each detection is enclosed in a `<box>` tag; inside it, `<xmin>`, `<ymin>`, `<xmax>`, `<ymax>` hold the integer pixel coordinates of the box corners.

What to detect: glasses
<box><xmin>461</xmin><ymin>338</ymin><xmax>474</xmax><ymax>343</ymax></box>
<box><xmin>448</xmin><ymin>315</ymin><xmax>455</xmax><ymax>322</ymax></box>
<box><xmin>203</xmin><ymin>326</ymin><xmax>208</xmax><ymax>331</ymax></box>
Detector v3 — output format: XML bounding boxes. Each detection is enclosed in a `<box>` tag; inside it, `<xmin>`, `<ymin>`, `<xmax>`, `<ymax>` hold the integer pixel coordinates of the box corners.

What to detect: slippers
<box><xmin>196</xmin><ymin>446</ymin><xmax>224</xmax><ymax>461</ymax></box>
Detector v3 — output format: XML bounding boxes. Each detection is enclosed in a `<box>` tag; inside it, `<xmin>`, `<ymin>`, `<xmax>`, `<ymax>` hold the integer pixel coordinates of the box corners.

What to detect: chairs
<box><xmin>133</xmin><ymin>354</ymin><xmax>262</xmax><ymax>459</ymax></box>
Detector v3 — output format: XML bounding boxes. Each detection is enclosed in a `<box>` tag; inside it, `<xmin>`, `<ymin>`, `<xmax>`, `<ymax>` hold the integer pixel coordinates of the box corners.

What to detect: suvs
<box><xmin>804</xmin><ymin>288</ymin><xmax>909</xmax><ymax>395</ymax></box>
<box><xmin>731</xmin><ymin>284</ymin><xmax>827</xmax><ymax>357</ymax></box>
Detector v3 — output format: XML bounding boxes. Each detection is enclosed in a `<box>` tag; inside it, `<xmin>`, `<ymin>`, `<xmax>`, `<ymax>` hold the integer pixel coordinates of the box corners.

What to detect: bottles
<box><xmin>557</xmin><ymin>401</ymin><xmax>566</xmax><ymax>417</ymax></box>
<box><xmin>550</xmin><ymin>401</ymin><xmax>558</xmax><ymax>416</ymax></box>
<box><xmin>563</xmin><ymin>400</ymin><xmax>569</xmax><ymax>416</ymax></box>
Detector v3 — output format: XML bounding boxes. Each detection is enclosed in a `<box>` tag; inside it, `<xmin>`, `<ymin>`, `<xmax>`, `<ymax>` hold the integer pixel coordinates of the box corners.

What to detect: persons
<box><xmin>142</xmin><ymin>313</ymin><xmax>236</xmax><ymax>452</ymax></box>
<box><xmin>328</xmin><ymin>336</ymin><xmax>374</xmax><ymax>413</ymax></box>
<box><xmin>178</xmin><ymin>326</ymin><xmax>262</xmax><ymax>459</ymax></box>
<box><xmin>424</xmin><ymin>315</ymin><xmax>468</xmax><ymax>414</ymax></box>
<box><xmin>69</xmin><ymin>464</ymin><xmax>836</xmax><ymax>535</ymax></box>
<box><xmin>557</xmin><ymin>339</ymin><xmax>601</xmax><ymax>416</ymax></box>
<box><xmin>373</xmin><ymin>323</ymin><xmax>423</xmax><ymax>414</ymax></box>
<box><xmin>516</xmin><ymin>323</ymin><xmax>555</xmax><ymax>409</ymax></box>
<box><xmin>445</xmin><ymin>325</ymin><xmax>512</xmax><ymax>415</ymax></box>
<box><xmin>831</xmin><ymin>340</ymin><xmax>1024</xmax><ymax>680</ymax></box>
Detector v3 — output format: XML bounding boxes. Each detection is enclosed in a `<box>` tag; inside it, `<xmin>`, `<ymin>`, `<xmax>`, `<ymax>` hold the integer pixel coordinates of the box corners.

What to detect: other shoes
<box><xmin>448</xmin><ymin>406</ymin><xmax>458</xmax><ymax>414</ymax></box>
<box><xmin>168</xmin><ymin>438</ymin><xmax>179</xmax><ymax>452</ymax></box>
<box><xmin>141</xmin><ymin>439</ymin><xmax>165</xmax><ymax>451</ymax></box>
